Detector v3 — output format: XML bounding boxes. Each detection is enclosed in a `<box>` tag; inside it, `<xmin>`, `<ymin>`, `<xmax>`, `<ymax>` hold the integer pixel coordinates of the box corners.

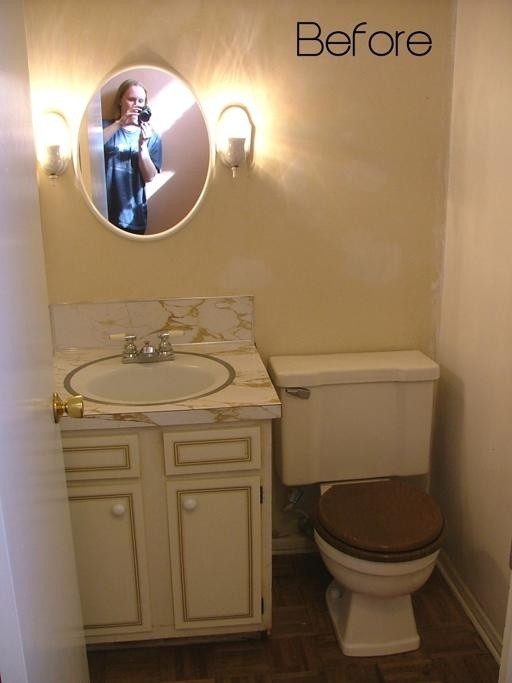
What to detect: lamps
<box><xmin>213</xmin><ymin>102</ymin><xmax>258</xmax><ymax>184</ymax></box>
<box><xmin>31</xmin><ymin>105</ymin><xmax>71</xmax><ymax>187</ymax></box>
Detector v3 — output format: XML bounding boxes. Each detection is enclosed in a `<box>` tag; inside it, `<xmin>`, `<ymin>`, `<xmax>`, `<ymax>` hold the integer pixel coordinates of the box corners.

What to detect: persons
<box><xmin>101</xmin><ymin>79</ymin><xmax>162</xmax><ymax>234</ymax></box>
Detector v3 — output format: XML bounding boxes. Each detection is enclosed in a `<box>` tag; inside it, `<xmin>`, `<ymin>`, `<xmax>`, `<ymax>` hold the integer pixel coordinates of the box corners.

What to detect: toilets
<box><xmin>268</xmin><ymin>348</ymin><xmax>448</xmax><ymax>655</ymax></box>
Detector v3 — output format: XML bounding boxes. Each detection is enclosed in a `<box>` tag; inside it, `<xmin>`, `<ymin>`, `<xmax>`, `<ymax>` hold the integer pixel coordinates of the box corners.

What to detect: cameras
<box><xmin>139</xmin><ymin>105</ymin><xmax>152</xmax><ymax>122</ymax></box>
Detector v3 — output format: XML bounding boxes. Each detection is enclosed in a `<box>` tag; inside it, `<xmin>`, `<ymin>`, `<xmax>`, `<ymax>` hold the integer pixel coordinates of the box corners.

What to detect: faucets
<box><xmin>137</xmin><ymin>344</ymin><xmax>158</xmax><ymax>361</ymax></box>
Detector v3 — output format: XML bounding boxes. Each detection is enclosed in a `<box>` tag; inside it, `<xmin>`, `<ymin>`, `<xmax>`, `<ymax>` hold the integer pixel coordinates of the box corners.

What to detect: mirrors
<box><xmin>71</xmin><ymin>63</ymin><xmax>213</xmax><ymax>246</ymax></box>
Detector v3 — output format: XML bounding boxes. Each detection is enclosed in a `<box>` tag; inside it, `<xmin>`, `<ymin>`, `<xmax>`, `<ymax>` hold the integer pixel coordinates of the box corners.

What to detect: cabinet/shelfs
<box><xmin>60</xmin><ymin>417</ymin><xmax>285</xmax><ymax>648</ymax></box>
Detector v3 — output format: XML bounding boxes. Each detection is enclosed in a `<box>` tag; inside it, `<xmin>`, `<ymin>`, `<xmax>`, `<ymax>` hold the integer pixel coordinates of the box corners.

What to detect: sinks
<box><xmin>82</xmin><ymin>362</ymin><xmax>217</xmax><ymax>404</ymax></box>
